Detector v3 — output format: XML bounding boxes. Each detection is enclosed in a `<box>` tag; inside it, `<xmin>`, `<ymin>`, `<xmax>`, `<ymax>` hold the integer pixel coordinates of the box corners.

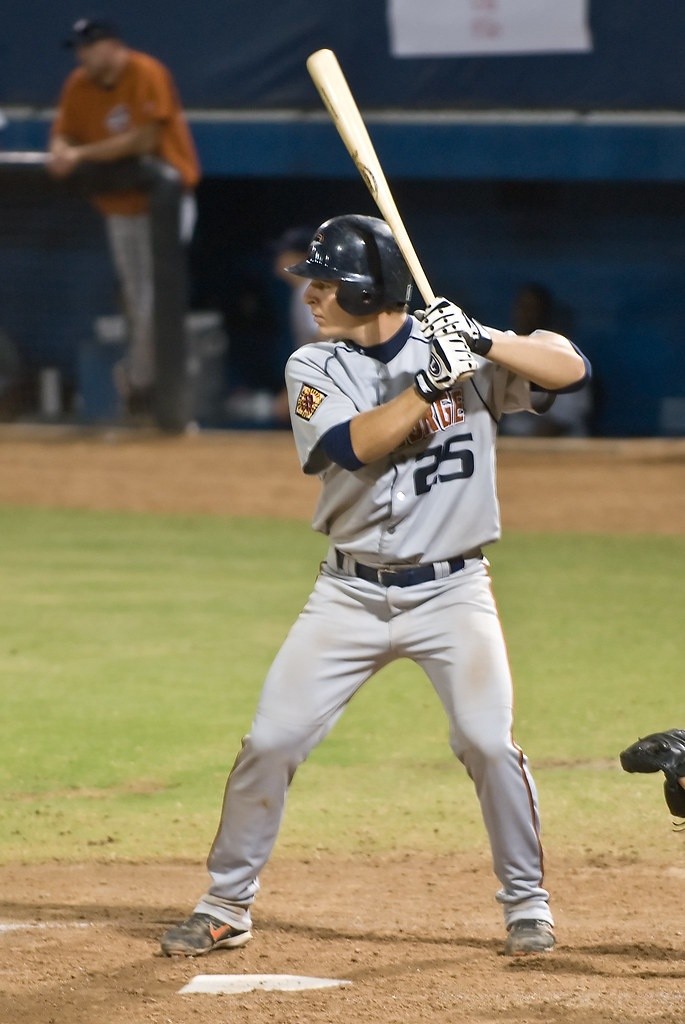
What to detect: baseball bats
<box><xmin>306</xmin><ymin>49</ymin><xmax>477</xmax><ymax>383</ymax></box>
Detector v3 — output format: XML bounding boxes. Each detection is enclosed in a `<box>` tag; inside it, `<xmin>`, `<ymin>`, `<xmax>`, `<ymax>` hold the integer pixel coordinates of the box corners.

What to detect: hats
<box><xmin>57</xmin><ymin>16</ymin><xmax>116</xmax><ymax>45</ymax></box>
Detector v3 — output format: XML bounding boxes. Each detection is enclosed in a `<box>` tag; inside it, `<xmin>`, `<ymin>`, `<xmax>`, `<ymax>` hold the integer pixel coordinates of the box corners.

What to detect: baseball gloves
<box><xmin>619</xmin><ymin>727</ymin><xmax>685</xmax><ymax>817</ymax></box>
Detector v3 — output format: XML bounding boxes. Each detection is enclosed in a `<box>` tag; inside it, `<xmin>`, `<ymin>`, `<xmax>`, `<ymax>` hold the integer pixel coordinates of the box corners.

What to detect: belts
<box><xmin>336</xmin><ymin>549</ymin><xmax>465</xmax><ymax>587</ymax></box>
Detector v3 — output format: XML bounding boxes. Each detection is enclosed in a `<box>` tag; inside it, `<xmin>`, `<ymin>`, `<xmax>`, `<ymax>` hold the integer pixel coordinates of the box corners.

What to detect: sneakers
<box><xmin>159</xmin><ymin>912</ymin><xmax>253</xmax><ymax>957</ymax></box>
<box><xmin>503</xmin><ymin>918</ymin><xmax>555</xmax><ymax>955</ymax></box>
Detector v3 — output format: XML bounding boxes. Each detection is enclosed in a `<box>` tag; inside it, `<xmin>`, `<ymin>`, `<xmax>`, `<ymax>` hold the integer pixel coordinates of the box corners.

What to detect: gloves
<box><xmin>413</xmin><ymin>295</ymin><xmax>493</xmax><ymax>357</ymax></box>
<box><xmin>413</xmin><ymin>333</ymin><xmax>479</xmax><ymax>403</ymax></box>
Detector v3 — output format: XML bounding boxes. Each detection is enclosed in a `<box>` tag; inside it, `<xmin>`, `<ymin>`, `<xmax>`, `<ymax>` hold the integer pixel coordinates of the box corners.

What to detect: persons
<box><xmin>51</xmin><ymin>14</ymin><xmax>199</xmax><ymax>400</ymax></box>
<box><xmin>158</xmin><ymin>215</ymin><xmax>593</xmax><ymax>959</ymax></box>
<box><xmin>502</xmin><ymin>281</ymin><xmax>588</xmax><ymax>437</ymax></box>
<box><xmin>226</xmin><ymin>228</ymin><xmax>329</xmax><ymax>419</ymax></box>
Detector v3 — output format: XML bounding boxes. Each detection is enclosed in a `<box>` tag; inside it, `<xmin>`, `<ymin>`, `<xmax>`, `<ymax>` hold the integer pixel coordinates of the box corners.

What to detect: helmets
<box><xmin>282</xmin><ymin>214</ymin><xmax>411</xmax><ymax>316</ymax></box>
